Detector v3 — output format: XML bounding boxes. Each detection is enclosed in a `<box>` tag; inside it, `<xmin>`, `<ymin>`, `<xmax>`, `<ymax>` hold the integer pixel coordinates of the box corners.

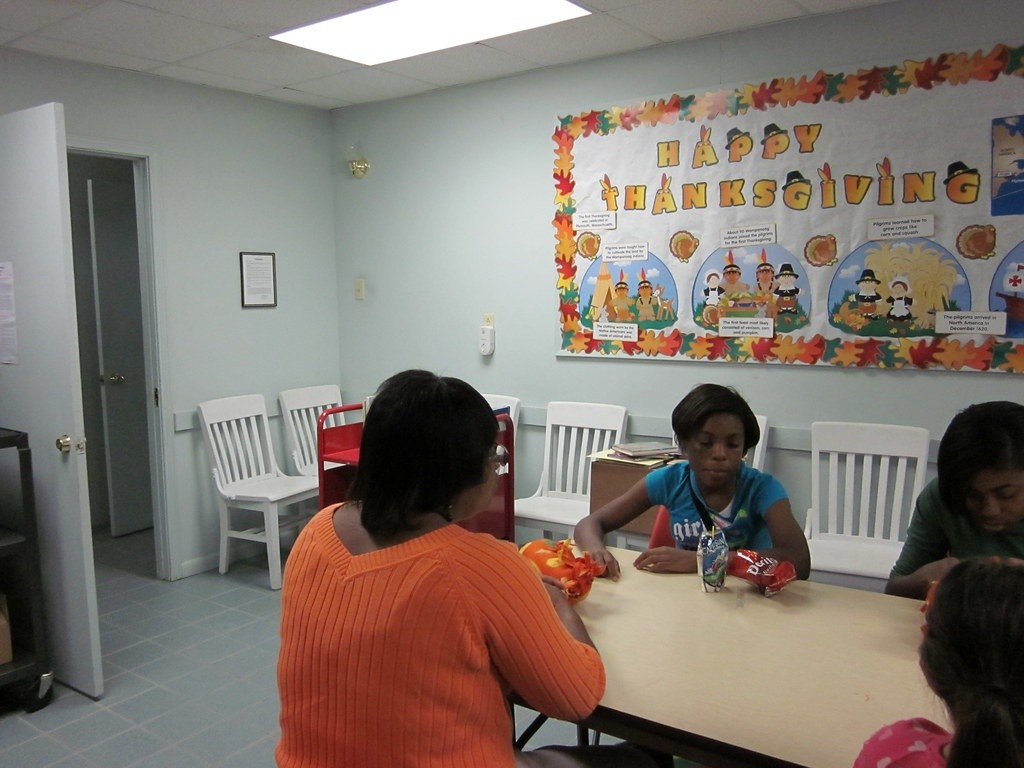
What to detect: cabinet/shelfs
<box><xmin>0</xmin><ymin>427</ymin><xmax>54</xmax><ymax>714</ymax></box>
<box><xmin>316</xmin><ymin>403</ymin><xmax>515</xmax><ymax>543</ymax></box>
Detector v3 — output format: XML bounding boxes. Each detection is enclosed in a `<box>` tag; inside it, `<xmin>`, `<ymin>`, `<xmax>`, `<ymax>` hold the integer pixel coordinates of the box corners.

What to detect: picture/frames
<box><xmin>239</xmin><ymin>251</ymin><xmax>277</xmax><ymax>308</ymax></box>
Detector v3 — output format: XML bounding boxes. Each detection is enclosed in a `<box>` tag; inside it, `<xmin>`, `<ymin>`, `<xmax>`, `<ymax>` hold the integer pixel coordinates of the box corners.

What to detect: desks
<box><xmin>507</xmin><ymin>545</ymin><xmax>954</xmax><ymax>768</ymax></box>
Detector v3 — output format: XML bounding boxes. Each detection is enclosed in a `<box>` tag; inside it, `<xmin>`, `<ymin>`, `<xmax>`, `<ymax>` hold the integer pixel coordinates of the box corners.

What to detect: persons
<box><xmin>573</xmin><ymin>383</ymin><xmax>811</xmax><ymax>580</ymax></box>
<box><xmin>854</xmin><ymin>557</ymin><xmax>1024</xmax><ymax>768</ymax></box>
<box><xmin>884</xmin><ymin>401</ymin><xmax>1024</xmax><ymax>600</ymax></box>
<box><xmin>274</xmin><ymin>368</ymin><xmax>674</xmax><ymax>768</ymax></box>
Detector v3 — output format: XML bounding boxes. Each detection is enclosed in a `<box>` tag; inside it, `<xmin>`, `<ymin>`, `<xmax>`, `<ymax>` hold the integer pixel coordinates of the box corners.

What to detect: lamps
<box><xmin>347</xmin><ymin>144</ymin><xmax>370</xmax><ymax>178</ymax></box>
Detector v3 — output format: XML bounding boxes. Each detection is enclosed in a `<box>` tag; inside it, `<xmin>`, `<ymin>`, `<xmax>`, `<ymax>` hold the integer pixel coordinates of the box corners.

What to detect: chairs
<box><xmin>197</xmin><ymin>384</ymin><xmax>350</xmax><ymax>589</ymax></box>
<box><xmin>514</xmin><ymin>401</ymin><xmax>628</xmax><ymax>539</ymax></box>
<box><xmin>804</xmin><ymin>421</ymin><xmax>930</xmax><ymax>580</ymax></box>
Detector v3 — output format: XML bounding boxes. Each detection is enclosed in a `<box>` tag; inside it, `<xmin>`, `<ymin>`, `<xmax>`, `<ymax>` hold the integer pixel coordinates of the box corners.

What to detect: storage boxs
<box><xmin>590</xmin><ymin>460</ymin><xmax>653</xmax><ymax>536</ymax></box>
